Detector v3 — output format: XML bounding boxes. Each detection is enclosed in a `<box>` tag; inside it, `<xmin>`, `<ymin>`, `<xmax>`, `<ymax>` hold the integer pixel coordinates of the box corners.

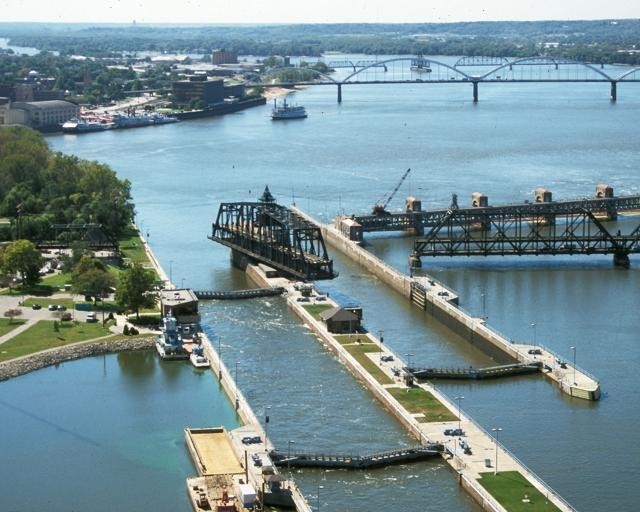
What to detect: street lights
<box><xmin>570</xmin><ymin>346</ymin><xmax>576</xmax><ymax>385</ymax></box>
<box><xmin>317</xmin><ymin>485</ymin><xmax>324</xmax><ymax>511</ymax></box>
<box><xmin>530</xmin><ymin>323</ymin><xmax>537</xmax><ymax>359</ymax></box>
<box><xmin>439</xmin><ymin>268</ymin><xmax>444</xmax><ymax>300</ymax></box>
<box><xmin>287</xmin><ymin>440</ymin><xmax>296</xmax><ymax>483</ymax></box>
<box><xmin>377</xmin><ymin>328</ymin><xmax>416</xmax><ymax>386</ymax></box>
<box><xmin>479</xmin><ymin>293</ymin><xmax>487</xmax><ymax>320</ymax></box>
<box><xmin>453</xmin><ymin>395</ymin><xmax>504</xmax><ymax>477</ymax></box>
<box><xmin>218</xmin><ymin>336</ymin><xmax>272</xmax><ymax>451</ymax></box>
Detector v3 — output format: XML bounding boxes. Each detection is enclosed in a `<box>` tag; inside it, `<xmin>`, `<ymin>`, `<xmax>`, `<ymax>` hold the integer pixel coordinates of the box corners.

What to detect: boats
<box><xmin>410</xmin><ymin>57</ymin><xmax>433</xmax><ymax>73</ymax></box>
<box><xmin>268</xmin><ymin>97</ymin><xmax>308</xmax><ymax>120</ymax></box>
<box><xmin>190</xmin><ymin>346</ymin><xmax>211</xmax><ymax>367</ymax></box>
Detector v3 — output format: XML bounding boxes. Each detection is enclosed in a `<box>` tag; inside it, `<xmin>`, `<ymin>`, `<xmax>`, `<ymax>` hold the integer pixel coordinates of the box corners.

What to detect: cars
<box><xmin>49</xmin><ymin>305</ymin><xmax>68</xmax><ymax>311</ymax></box>
<box><xmin>38</xmin><ymin>261</ymin><xmax>65</xmax><ymax>276</ymax></box>
<box><xmin>32</xmin><ymin>304</ymin><xmax>41</xmax><ymax>309</ymax></box>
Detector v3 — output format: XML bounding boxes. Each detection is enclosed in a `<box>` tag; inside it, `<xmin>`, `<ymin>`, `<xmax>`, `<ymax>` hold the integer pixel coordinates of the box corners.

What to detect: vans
<box><xmin>87</xmin><ymin>312</ymin><xmax>96</xmax><ymax>321</ymax></box>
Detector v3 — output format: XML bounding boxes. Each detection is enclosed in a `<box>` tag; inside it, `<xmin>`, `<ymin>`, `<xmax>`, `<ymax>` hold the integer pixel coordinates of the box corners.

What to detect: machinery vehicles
<box><xmin>373</xmin><ymin>167</ymin><xmax>412</xmax><ymax>214</ymax></box>
